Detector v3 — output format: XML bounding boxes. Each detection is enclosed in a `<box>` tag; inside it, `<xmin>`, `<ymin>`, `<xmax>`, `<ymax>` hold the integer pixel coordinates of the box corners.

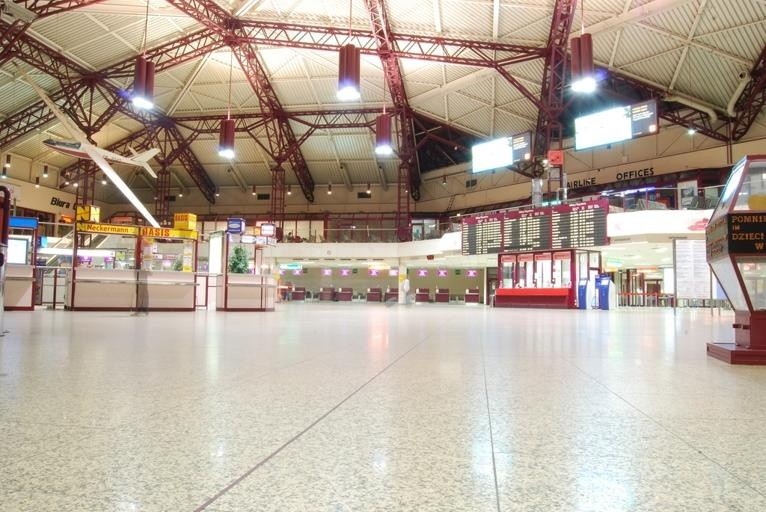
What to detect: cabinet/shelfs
<box><xmin>3</xmin><ymin>264</ymin><xmax>36</xmax><ymax>311</ymax></box>
<box><xmin>64</xmin><ymin>269</ymin><xmax>209</xmax><ymax>311</ymax></box>
<box><xmin>493</xmin><ymin>248</ymin><xmax>601</xmax><ymax>309</ymax></box>
<box><xmin>216</xmin><ymin>273</ymin><xmax>277</xmax><ymax>312</ymax></box>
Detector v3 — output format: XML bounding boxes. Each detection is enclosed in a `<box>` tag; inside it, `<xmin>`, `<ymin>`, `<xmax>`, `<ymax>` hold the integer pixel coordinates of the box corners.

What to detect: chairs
<box><xmin>279</xmin><ymin>280</ymin><xmax>479</xmax><ymax>303</ymax></box>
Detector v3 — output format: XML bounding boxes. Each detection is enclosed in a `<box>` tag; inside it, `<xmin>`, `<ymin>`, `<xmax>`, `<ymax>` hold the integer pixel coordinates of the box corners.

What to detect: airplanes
<box><xmin>9</xmin><ymin>60</ymin><xmax>161</xmax><ymax>229</ymax></box>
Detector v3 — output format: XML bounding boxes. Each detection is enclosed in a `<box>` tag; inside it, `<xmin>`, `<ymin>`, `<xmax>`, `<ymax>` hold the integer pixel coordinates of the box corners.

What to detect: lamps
<box><xmin>219</xmin><ymin>44</ymin><xmax>235</xmax><ymax>160</ymax></box>
<box><xmin>687</xmin><ymin>45</ymin><xmax>696</xmax><ymax>136</ymax></box>
<box><xmin>339</xmin><ymin>0</ymin><xmax>361</xmax><ymax>96</ymax></box>
<box><xmin>133</xmin><ymin>1</ymin><xmax>155</xmax><ymax>110</ymax></box>
<box><xmin>375</xmin><ymin>61</ymin><xmax>392</xmax><ymax>157</ymax></box>
<box><xmin>571</xmin><ymin>1</ymin><xmax>598</xmax><ymax>95</ymax></box>
